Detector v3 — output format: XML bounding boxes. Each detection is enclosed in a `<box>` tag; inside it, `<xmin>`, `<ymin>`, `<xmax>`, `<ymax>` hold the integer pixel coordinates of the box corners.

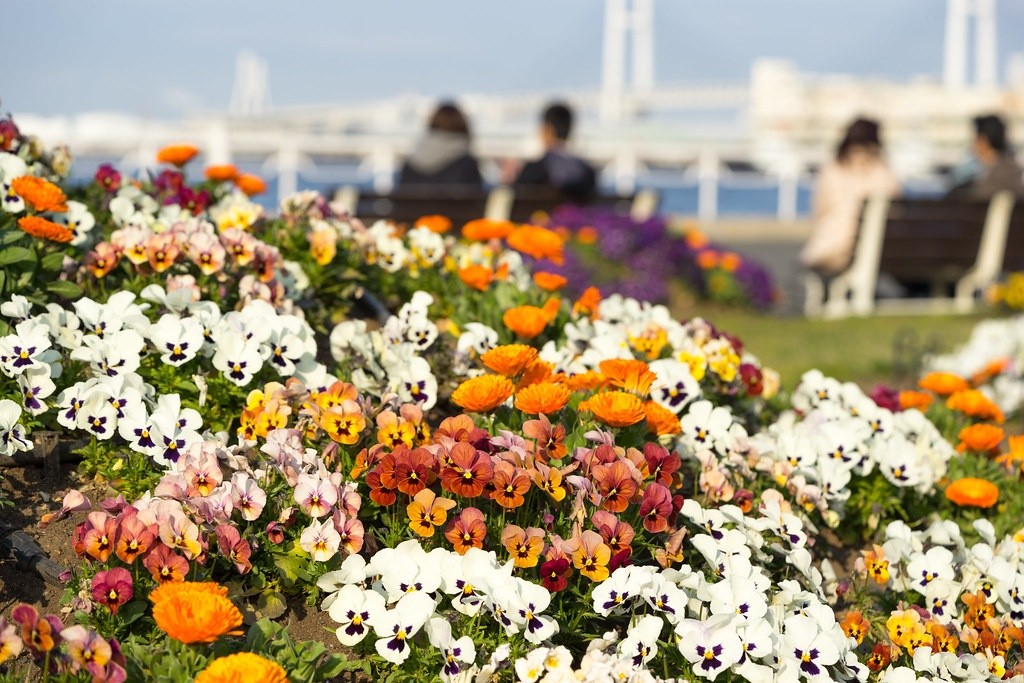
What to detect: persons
<box><xmin>796</xmin><ymin>114</ymin><xmax>903</xmax><ymax>271</ymax></box>
<box><xmin>510</xmin><ymin>103</ymin><xmax>598</xmax><ymax>223</ymax></box>
<box><xmin>948</xmin><ymin>114</ymin><xmax>1024</xmax><ymax>200</ymax></box>
<box><xmin>393</xmin><ymin>102</ymin><xmax>487</xmax><ymax>237</ymax></box>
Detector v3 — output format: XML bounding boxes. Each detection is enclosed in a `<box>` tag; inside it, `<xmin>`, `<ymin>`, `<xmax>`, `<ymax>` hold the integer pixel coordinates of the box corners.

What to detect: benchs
<box><xmin>803</xmin><ymin>194</ymin><xmax>1024</xmax><ymax>323</ymax></box>
<box><xmin>321</xmin><ymin>178</ymin><xmax>635</xmax><ymax>241</ymax></box>
